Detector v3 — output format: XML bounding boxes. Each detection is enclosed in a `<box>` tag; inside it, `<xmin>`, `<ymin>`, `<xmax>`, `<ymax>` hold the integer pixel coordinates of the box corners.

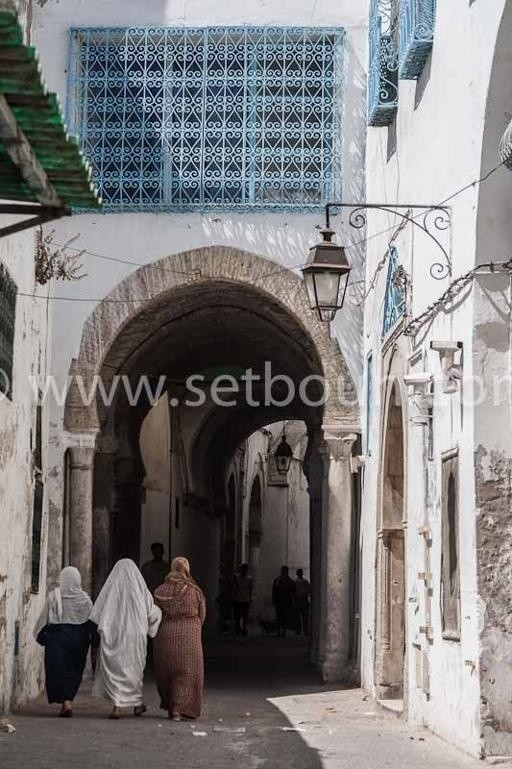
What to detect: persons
<box><xmin>149</xmin><ymin>558</ymin><xmax>207</xmax><ymax>725</ymax></box>
<box><xmin>138</xmin><ymin>543</ymin><xmax>168</xmax><ymax>589</ymax></box>
<box><xmin>216</xmin><ymin>558</ymin><xmax>253</xmax><ymax>637</ymax></box>
<box><xmin>31</xmin><ymin>567</ymin><xmax>96</xmax><ymax>718</ymax></box>
<box><xmin>88</xmin><ymin>558</ymin><xmax>164</xmax><ymax>719</ymax></box>
<box><xmin>270</xmin><ymin>566</ymin><xmax>298</xmax><ymax>640</ymax></box>
<box><xmin>293</xmin><ymin>568</ymin><xmax>311</xmax><ymax>637</ymax></box>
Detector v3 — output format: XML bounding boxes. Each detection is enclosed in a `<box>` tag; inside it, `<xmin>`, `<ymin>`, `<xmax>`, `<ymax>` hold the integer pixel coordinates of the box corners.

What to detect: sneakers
<box><xmin>58</xmin><ymin>701</ymin><xmax>72</xmax><ymax>718</ymax></box>
<box><xmin>168</xmin><ymin>711</ymin><xmax>180</xmax><ymax>722</ymax></box>
<box><xmin>134</xmin><ymin>705</ymin><xmax>147</xmax><ymax>717</ymax></box>
<box><xmin>109</xmin><ymin>706</ymin><xmax>120</xmax><ymax>719</ymax></box>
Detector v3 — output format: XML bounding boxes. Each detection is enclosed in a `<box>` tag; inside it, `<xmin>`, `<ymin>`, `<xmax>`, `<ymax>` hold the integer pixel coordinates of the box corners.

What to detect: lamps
<box><xmin>300</xmin><ymin>201</ymin><xmax>452</xmax><ymax>326</ymax></box>
<box><xmin>274</xmin><ymin>420</ymin><xmax>294</xmax><ymax>476</ymax></box>
